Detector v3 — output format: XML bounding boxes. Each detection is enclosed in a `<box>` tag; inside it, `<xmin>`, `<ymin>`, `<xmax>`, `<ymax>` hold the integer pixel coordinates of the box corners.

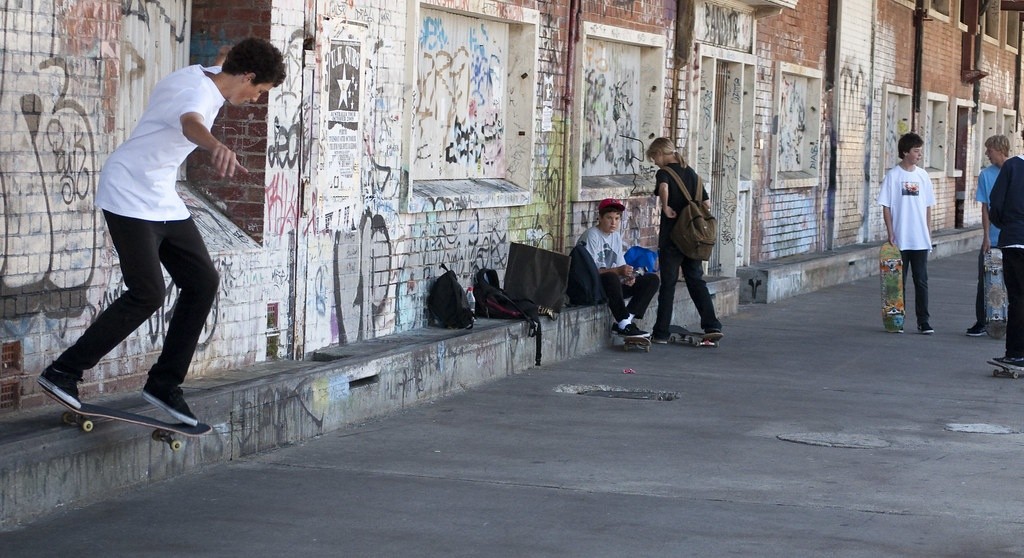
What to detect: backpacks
<box><xmin>660</xmin><ymin>165</ymin><xmax>717</xmax><ymax>261</ymax></box>
<box><xmin>474</xmin><ymin>266</ymin><xmax>542</xmax><ymax>366</ymax></box>
<box><xmin>624</xmin><ymin>246</ymin><xmax>661</xmax><ymax>275</ymax></box>
<box><xmin>427</xmin><ymin>263</ymin><xmax>478</xmax><ymax>331</ymax></box>
<box><xmin>568</xmin><ymin>241</ymin><xmax>609</xmax><ymax>305</ymax></box>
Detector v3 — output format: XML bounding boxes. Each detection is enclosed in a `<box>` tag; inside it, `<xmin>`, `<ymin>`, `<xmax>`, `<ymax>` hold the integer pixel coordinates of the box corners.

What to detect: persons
<box><xmin>577</xmin><ymin>198</ymin><xmax>660</xmax><ymax>337</ymax></box>
<box><xmin>645</xmin><ymin>138</ymin><xmax>722</xmax><ymax>344</ymax></box>
<box><xmin>878</xmin><ymin>132</ymin><xmax>936</xmax><ymax>334</ymax></box>
<box><xmin>965</xmin><ymin>135</ymin><xmax>1010</xmax><ymax>336</ymax></box>
<box><xmin>988</xmin><ymin>129</ymin><xmax>1024</xmax><ymax>371</ymax></box>
<box><xmin>37</xmin><ymin>38</ymin><xmax>287</xmax><ymax>427</ymax></box>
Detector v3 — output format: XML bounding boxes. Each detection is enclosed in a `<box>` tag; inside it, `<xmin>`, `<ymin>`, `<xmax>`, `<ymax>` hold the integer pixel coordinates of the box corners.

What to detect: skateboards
<box><xmin>879</xmin><ymin>240</ymin><xmax>906</xmax><ymax>334</ymax></box>
<box><xmin>985</xmin><ymin>359</ymin><xmax>1024</xmax><ymax>380</ymax></box>
<box><xmin>622</xmin><ymin>336</ymin><xmax>653</xmax><ymax>354</ymax></box>
<box><xmin>667</xmin><ymin>325</ymin><xmax>724</xmax><ymax>349</ymax></box>
<box><xmin>984</xmin><ymin>247</ymin><xmax>1008</xmax><ymax>341</ymax></box>
<box><xmin>38</xmin><ymin>387</ymin><xmax>213</xmax><ymax>452</ymax></box>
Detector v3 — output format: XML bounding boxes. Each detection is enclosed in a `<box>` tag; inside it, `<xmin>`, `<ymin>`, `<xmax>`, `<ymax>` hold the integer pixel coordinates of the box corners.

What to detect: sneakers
<box><xmin>898</xmin><ymin>327</ymin><xmax>904</xmax><ymax>333</ymax></box>
<box><xmin>966</xmin><ymin>321</ymin><xmax>987</xmax><ymax>336</ymax></box>
<box><xmin>610</xmin><ymin>321</ymin><xmax>650</xmax><ymax>339</ymax></box>
<box><xmin>917</xmin><ymin>322</ymin><xmax>934</xmax><ymax>334</ymax></box>
<box><xmin>141</xmin><ymin>378</ymin><xmax>198</xmax><ymax>427</ymax></box>
<box><xmin>37</xmin><ymin>364</ymin><xmax>84</xmax><ymax>410</ymax></box>
<box><xmin>990</xmin><ymin>354</ymin><xmax>1024</xmax><ymax>370</ymax></box>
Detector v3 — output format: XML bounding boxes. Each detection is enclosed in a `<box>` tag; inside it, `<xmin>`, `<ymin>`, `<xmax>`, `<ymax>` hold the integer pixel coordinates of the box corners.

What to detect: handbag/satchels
<box><xmin>503</xmin><ymin>232</ymin><xmax>572</xmax><ymax>314</ymax></box>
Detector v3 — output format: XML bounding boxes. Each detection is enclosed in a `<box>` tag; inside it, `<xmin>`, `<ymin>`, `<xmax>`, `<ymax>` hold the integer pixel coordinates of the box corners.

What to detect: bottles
<box><xmin>466</xmin><ymin>287</ymin><xmax>476</xmax><ymax>316</ymax></box>
<box><xmin>620</xmin><ymin>266</ymin><xmax>648</xmax><ymax>285</ymax></box>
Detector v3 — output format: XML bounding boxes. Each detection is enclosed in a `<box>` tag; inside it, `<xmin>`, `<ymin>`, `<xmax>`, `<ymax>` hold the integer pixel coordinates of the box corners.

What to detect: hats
<box><xmin>599</xmin><ymin>198</ymin><xmax>625</xmax><ymax>212</ymax></box>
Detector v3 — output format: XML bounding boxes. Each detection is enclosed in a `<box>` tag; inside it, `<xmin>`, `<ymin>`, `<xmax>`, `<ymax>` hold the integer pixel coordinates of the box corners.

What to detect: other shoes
<box><xmin>704</xmin><ymin>328</ymin><xmax>722</xmax><ymax>333</ymax></box>
<box><xmin>651</xmin><ymin>335</ymin><xmax>668</xmax><ymax>344</ymax></box>
